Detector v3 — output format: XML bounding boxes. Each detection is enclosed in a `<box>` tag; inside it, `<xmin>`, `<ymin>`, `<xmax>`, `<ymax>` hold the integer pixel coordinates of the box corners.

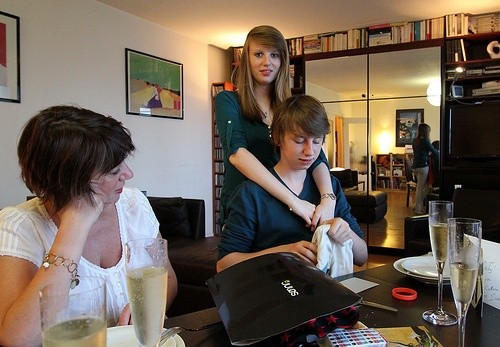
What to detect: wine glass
<box><xmin>447</xmin><ymin>218</ymin><xmax>482</xmax><ymax>346</ymax></box>
<box><xmin>422</xmin><ymin>200</ymin><xmax>458</xmax><ymax>325</ymax></box>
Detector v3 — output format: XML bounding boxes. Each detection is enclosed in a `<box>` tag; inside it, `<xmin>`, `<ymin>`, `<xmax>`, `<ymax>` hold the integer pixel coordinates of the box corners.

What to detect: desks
<box><xmin>164</xmin><ymin>235</ymin><xmax>500</xmax><ymax>347</ymax></box>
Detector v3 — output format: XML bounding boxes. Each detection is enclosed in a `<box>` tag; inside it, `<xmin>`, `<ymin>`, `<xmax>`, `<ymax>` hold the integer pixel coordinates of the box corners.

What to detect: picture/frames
<box><xmin>125</xmin><ymin>47</ymin><xmax>184</xmax><ymax>120</ymax></box>
<box><xmin>396</xmin><ymin>109</ymin><xmax>425</xmax><ymax>147</ymax></box>
<box><xmin>0</xmin><ymin>11</ymin><xmax>26</xmax><ymax>103</ymax></box>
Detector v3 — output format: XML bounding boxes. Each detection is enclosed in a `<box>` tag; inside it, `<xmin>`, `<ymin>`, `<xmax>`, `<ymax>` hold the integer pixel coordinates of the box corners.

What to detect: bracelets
<box><xmin>42</xmin><ymin>254</ymin><xmax>80</xmax><ymax>289</ymax></box>
<box><xmin>290</xmin><ymin>196</ymin><xmax>297</xmax><ymax>210</ymax></box>
<box><xmin>321</xmin><ymin>193</ymin><xmax>336</xmax><ymax>200</ymax></box>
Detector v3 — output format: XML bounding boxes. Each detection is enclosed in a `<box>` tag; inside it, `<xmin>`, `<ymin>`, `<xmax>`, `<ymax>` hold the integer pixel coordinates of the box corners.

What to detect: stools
<box><xmin>343</xmin><ymin>191</ymin><xmax>387</xmax><ymax>222</ymax></box>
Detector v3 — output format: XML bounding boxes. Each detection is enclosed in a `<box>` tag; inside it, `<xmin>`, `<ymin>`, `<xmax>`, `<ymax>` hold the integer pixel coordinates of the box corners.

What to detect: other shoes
<box><xmin>413</xmin><ymin>207</ymin><xmax>427</xmax><ymax>216</ymax></box>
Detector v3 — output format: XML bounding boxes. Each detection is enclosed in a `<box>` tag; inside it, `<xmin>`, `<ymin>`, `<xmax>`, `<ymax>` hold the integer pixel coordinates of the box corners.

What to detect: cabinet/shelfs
<box><xmin>212</xmin><ymin>83</ymin><xmax>224</xmax><ymax>234</ymax></box>
<box><xmin>374</xmin><ymin>153</ymin><xmax>415</xmax><ymax>193</ymax></box>
<box><xmin>445</xmin><ymin>32</ymin><xmax>500</xmax><ymax>101</ymax></box>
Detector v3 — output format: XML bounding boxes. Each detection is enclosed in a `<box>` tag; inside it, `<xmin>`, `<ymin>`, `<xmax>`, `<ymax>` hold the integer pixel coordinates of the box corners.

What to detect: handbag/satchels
<box><xmin>205</xmin><ymin>252</ymin><xmax>363</xmax><ymax>347</ymax></box>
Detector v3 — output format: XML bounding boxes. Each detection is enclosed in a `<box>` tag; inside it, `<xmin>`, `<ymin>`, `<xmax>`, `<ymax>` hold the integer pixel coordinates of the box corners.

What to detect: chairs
<box><xmin>402</xmin><ymin>156</ymin><xmax>419</xmax><ymax>206</ymax></box>
<box><xmin>147</xmin><ymin>197</ymin><xmax>221</xmax><ymax>317</ymax></box>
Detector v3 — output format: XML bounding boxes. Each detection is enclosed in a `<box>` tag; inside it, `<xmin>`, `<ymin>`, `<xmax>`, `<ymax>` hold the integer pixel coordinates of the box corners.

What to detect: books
<box><xmin>211</xmin><ymin>85</ymin><xmax>225</xmax><ymax>235</ymax></box>
<box><xmin>232</xmin><ymin>12</ymin><xmax>500</xmax><ymax>96</ymax></box>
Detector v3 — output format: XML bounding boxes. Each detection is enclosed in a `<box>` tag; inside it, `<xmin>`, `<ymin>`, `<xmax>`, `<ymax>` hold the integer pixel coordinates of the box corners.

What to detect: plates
<box><xmin>393</xmin><ymin>256</ymin><xmax>451</xmax><ymax>286</ymax></box>
<box><xmin>106</xmin><ymin>325</ymin><xmax>185</xmax><ymax>347</ymax></box>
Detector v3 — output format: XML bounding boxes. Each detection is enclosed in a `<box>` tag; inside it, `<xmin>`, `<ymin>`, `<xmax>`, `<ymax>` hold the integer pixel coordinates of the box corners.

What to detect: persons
<box><xmin>0</xmin><ymin>106</ymin><xmax>178</xmax><ymax>347</ymax></box>
<box><xmin>432</xmin><ymin>140</ymin><xmax>439</xmax><ymax>149</ymax></box>
<box><xmin>215</xmin><ymin>94</ymin><xmax>368</xmax><ymax>277</ymax></box>
<box><xmin>214</xmin><ymin>25</ymin><xmax>337</xmax><ymax>230</ymax></box>
<box><xmin>413</xmin><ymin>123</ymin><xmax>439</xmax><ymax>214</ymax></box>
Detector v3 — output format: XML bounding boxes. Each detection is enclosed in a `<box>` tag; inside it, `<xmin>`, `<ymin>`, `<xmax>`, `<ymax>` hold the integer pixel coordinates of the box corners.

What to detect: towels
<box><xmin>305</xmin><ymin>224</ymin><xmax>354</xmax><ymax>278</ymax></box>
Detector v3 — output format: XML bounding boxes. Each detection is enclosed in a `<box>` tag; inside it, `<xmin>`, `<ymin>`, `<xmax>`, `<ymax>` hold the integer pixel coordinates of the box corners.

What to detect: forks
<box><xmin>160</xmin><ymin>320</ymin><xmax>223</xmax><ymax>346</ymax></box>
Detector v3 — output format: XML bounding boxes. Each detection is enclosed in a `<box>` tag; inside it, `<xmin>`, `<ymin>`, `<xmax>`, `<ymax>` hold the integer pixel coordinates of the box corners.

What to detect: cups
<box><xmin>39</xmin><ymin>273</ymin><xmax>107</xmax><ymax>347</ymax></box>
<box><xmin>125</xmin><ymin>238</ymin><xmax>169</xmax><ymax>347</ymax></box>
